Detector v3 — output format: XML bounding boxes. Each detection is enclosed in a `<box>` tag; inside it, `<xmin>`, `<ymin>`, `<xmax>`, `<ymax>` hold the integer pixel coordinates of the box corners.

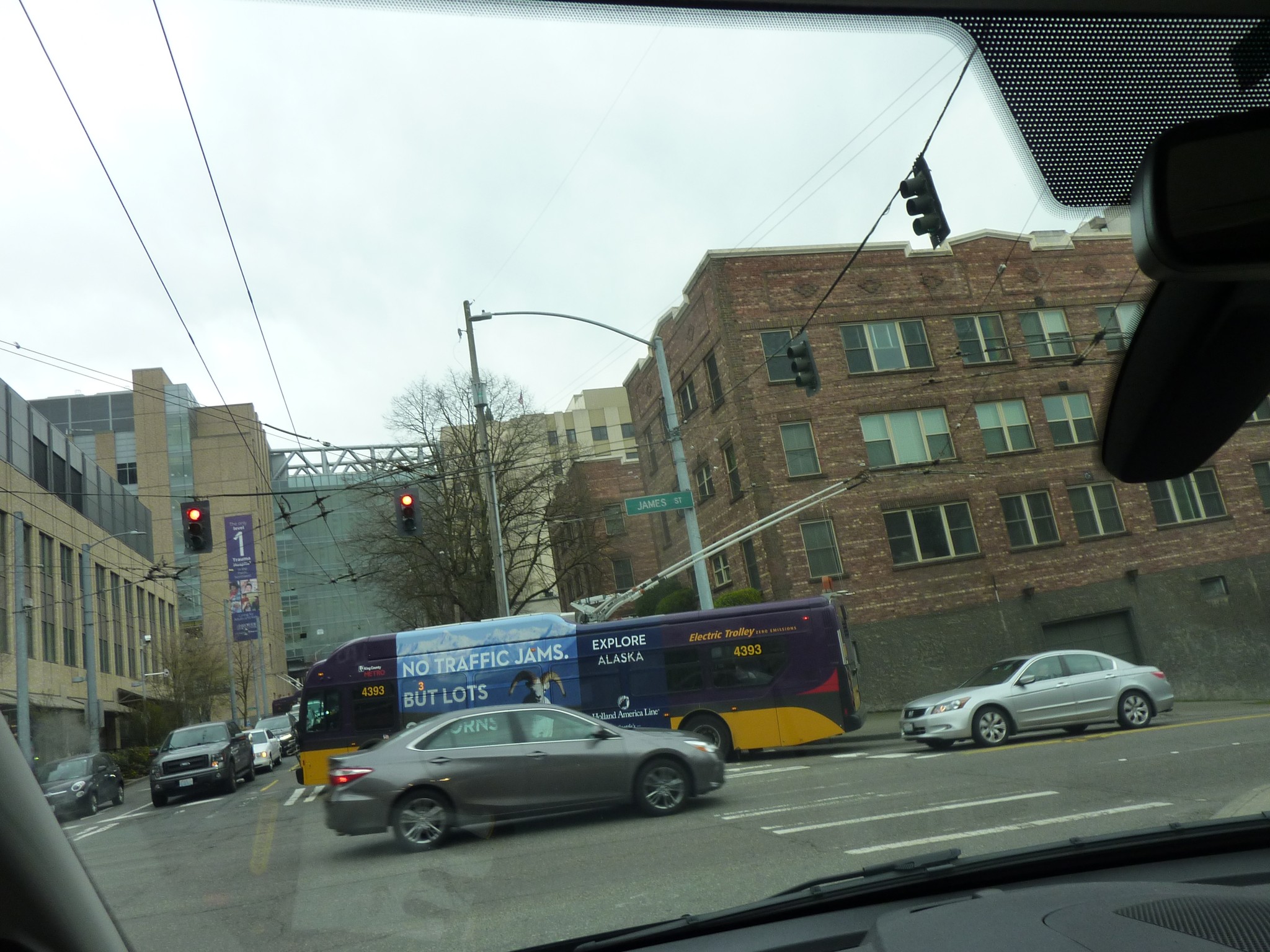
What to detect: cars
<box><xmin>900</xmin><ymin>647</ymin><xmax>1172</xmax><ymax>752</ymax></box>
<box><xmin>242</xmin><ymin>700</ymin><xmax>326</xmax><ymax>773</ymax></box>
<box><xmin>320</xmin><ymin>702</ymin><xmax>728</xmax><ymax>853</ymax></box>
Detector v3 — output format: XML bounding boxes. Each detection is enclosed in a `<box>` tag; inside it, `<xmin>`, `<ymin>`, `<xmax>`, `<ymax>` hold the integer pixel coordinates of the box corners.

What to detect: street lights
<box><xmin>82</xmin><ymin>530</ymin><xmax>148</xmax><ymax>752</ymax></box>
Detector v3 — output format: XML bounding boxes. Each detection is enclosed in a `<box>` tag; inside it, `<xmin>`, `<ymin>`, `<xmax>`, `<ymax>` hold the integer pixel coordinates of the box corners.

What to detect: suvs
<box><xmin>37</xmin><ymin>751</ymin><xmax>124</xmax><ymax>819</ymax></box>
<box><xmin>149</xmin><ymin>720</ymin><xmax>255</xmax><ymax>807</ymax></box>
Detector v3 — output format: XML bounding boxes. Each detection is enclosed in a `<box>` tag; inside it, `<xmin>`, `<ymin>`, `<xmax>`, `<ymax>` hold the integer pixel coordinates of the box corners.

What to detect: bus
<box><xmin>272</xmin><ymin>596</ymin><xmax>866</xmax><ymax>782</ymax></box>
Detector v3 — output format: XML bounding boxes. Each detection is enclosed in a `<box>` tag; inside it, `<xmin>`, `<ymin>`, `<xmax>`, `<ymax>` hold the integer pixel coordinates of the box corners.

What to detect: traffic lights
<box><xmin>394</xmin><ymin>487</ymin><xmax>422</xmax><ymax>537</ymax></box>
<box><xmin>181</xmin><ymin>499</ymin><xmax>213</xmax><ymax>555</ymax></box>
<box><xmin>788</xmin><ymin>340</ymin><xmax>821</xmax><ymax>394</ymax></box>
<box><xmin>899</xmin><ymin>159</ymin><xmax>951</xmax><ymax>249</ymax></box>
